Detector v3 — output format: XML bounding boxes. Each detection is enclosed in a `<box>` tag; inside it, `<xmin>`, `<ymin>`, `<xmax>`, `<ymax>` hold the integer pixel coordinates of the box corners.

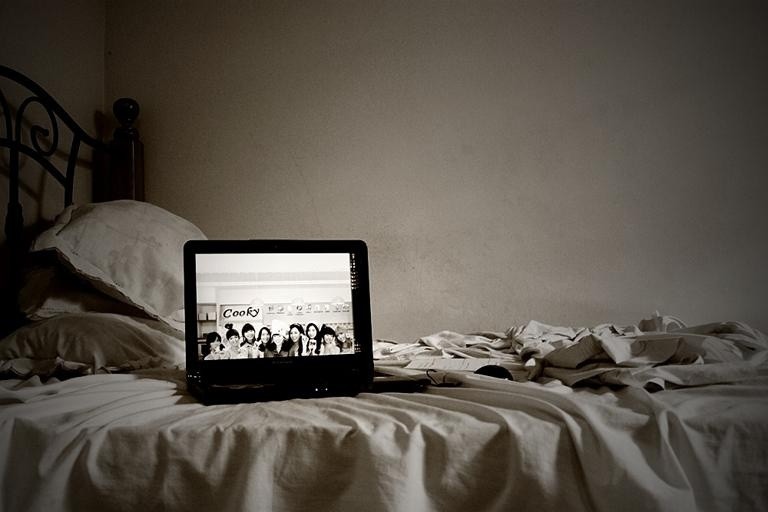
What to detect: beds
<box><xmin>2</xmin><ymin>62</ymin><xmax>767</xmax><ymax>512</ymax></box>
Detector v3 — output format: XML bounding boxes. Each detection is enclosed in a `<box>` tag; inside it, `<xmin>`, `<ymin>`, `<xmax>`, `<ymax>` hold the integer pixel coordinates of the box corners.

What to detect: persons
<box><xmin>202</xmin><ymin>322</ymin><xmax>354</xmax><ymax>362</ymax></box>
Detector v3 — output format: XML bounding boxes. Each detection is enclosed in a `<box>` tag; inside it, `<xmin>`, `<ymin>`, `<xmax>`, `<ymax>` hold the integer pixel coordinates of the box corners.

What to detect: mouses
<box><xmin>474</xmin><ymin>365</ymin><xmax>516</xmax><ymax>383</ymax></box>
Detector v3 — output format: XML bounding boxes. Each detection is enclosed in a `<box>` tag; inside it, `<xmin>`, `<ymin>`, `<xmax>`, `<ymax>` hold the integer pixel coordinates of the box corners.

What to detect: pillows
<box><xmin>1</xmin><ymin>198</ymin><xmax>211</xmax><ymax>379</ymax></box>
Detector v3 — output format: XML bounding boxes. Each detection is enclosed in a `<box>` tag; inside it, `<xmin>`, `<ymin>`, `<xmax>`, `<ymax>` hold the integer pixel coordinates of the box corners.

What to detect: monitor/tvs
<box><xmin>183</xmin><ymin>239</ymin><xmax>375</xmax><ymax>405</ymax></box>
